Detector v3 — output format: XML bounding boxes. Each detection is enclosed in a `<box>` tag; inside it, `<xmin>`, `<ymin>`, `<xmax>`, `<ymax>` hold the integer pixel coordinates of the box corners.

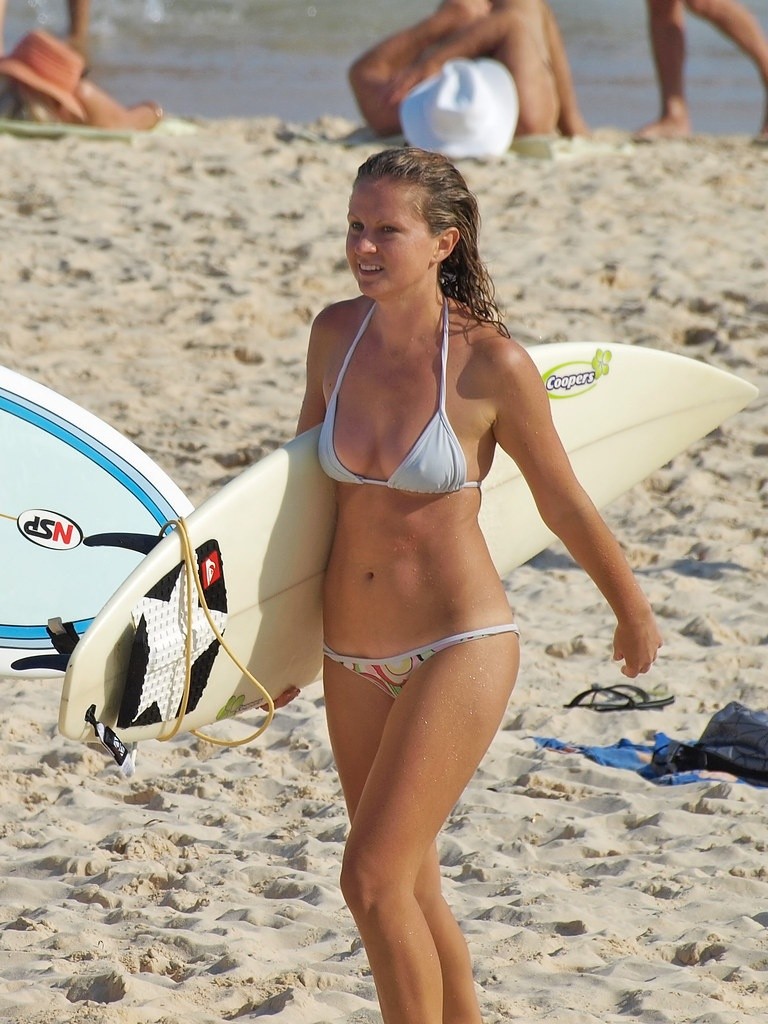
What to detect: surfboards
<box><xmin>0</xmin><ymin>363</ymin><xmax>199</xmax><ymax>653</ymax></box>
<box><xmin>57</xmin><ymin>338</ymin><xmax>762</xmax><ymax>748</ymax></box>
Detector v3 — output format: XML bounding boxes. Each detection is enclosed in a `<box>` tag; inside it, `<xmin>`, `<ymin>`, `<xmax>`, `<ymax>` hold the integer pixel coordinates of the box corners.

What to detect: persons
<box><xmin>252</xmin><ymin>149</ymin><xmax>663</xmax><ymax>1024</ymax></box>
<box><xmin>0</xmin><ymin>0</ymin><xmax>168</xmax><ymax>135</ymax></box>
<box><xmin>348</xmin><ymin>0</ymin><xmax>768</xmax><ymax>173</ymax></box>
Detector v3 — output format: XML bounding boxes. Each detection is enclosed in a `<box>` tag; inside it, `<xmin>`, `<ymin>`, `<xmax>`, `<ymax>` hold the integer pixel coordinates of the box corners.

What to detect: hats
<box><xmin>0</xmin><ymin>31</ymin><xmax>86</xmax><ymax>122</ymax></box>
<box><xmin>398</xmin><ymin>57</ymin><xmax>520</xmax><ymax>163</ymax></box>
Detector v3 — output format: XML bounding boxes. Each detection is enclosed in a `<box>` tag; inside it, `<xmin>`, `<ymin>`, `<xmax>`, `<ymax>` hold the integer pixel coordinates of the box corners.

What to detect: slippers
<box><xmin>564</xmin><ymin>683</ymin><xmax>674</xmax><ymax>711</ymax></box>
